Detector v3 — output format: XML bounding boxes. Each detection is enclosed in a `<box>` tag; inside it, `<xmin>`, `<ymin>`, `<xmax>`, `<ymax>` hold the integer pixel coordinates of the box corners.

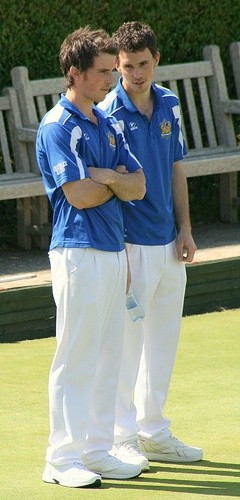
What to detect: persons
<box><xmin>35</xmin><ymin>24</ymin><xmax>146</xmax><ymax>488</ymax></box>
<box><xmin>96</xmin><ymin>22</ymin><xmax>204</xmax><ymax>473</ymax></box>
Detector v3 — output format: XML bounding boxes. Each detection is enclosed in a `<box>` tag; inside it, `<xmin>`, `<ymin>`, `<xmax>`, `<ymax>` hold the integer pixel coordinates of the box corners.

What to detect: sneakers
<box><xmin>43</xmin><ymin>462</ymin><xmax>102</xmax><ymax>488</ymax></box>
<box><xmin>137</xmin><ymin>435</ymin><xmax>203</xmax><ymax>462</ymax></box>
<box><xmin>85</xmin><ymin>456</ymin><xmax>143</xmax><ymax>479</ymax></box>
<box><xmin>108</xmin><ymin>439</ymin><xmax>150</xmax><ymax>471</ymax></box>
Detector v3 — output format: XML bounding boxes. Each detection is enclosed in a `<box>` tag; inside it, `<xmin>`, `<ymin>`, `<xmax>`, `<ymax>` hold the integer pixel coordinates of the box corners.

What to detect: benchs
<box><xmin>0</xmin><ymin>41</ymin><xmax>240</xmax><ymax>250</ymax></box>
